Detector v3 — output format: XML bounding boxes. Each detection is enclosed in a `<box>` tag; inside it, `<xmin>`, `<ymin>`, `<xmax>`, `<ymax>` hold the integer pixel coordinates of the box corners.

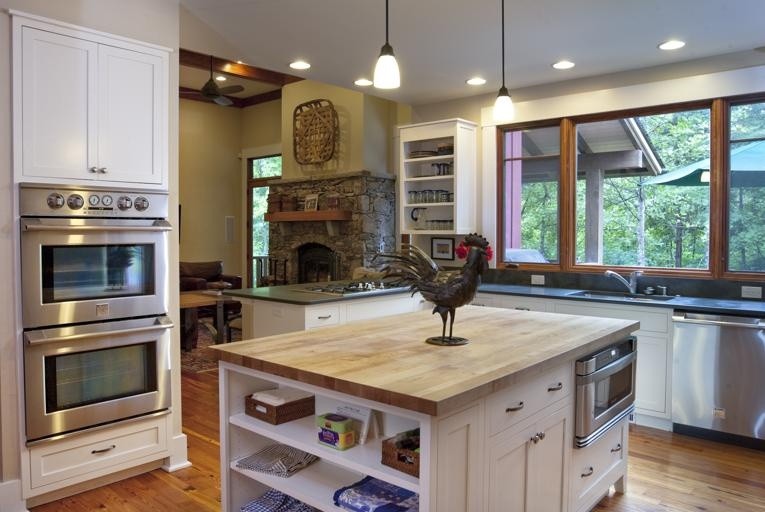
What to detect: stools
<box><xmin>227</xmin><ymin>314</ymin><xmax>242</xmax><ymax>343</ymax></box>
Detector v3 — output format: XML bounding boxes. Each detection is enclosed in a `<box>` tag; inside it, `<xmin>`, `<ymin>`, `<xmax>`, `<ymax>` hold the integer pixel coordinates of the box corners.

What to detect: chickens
<box><xmin>370</xmin><ymin>233</ymin><xmax>491</xmax><ymax>344</ymax></box>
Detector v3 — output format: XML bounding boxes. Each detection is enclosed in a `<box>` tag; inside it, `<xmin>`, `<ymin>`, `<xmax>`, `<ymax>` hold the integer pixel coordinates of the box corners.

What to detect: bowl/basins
<box><xmin>438</xmin><ymin>142</ymin><xmax>453</xmax><ymax>154</ymax></box>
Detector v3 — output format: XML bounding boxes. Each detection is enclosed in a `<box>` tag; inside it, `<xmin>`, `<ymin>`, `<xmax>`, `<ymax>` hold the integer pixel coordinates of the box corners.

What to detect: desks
<box><xmin>179</xmin><ymin>289</ymin><xmax>237</xmax><ymax>353</ymax></box>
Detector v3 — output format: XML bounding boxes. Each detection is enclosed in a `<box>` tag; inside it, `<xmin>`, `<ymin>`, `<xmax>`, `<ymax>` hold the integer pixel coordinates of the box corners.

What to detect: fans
<box><xmin>179</xmin><ymin>56</ymin><xmax>245</xmax><ymax>107</ymax></box>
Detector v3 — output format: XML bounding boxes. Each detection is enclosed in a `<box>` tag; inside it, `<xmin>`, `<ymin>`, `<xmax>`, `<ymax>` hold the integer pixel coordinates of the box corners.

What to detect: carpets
<box><xmin>180</xmin><ymin>316</ymin><xmax>242</xmax><ymax>376</ymax></box>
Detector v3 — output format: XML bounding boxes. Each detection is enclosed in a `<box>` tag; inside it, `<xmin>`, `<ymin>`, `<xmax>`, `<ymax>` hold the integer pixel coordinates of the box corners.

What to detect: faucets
<box><xmin>604</xmin><ymin>271</ymin><xmax>644</xmax><ymax>294</ymax></box>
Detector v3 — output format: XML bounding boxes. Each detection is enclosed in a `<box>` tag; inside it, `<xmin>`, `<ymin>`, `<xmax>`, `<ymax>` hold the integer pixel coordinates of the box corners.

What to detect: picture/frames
<box><xmin>303</xmin><ymin>193</ymin><xmax>319</xmax><ymax>212</ymax></box>
<box><xmin>430</xmin><ymin>237</ymin><xmax>455</xmax><ymax>260</ymax></box>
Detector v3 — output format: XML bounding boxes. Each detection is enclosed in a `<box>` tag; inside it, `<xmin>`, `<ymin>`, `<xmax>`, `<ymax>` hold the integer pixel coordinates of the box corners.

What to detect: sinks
<box><xmin>570</xmin><ymin>293</ymin><xmax>674</xmax><ymax>303</ymax></box>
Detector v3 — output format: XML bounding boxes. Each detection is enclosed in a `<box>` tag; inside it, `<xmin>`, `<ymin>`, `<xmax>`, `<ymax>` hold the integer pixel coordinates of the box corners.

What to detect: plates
<box><xmin>408</xmin><ymin>151</ymin><xmax>438</xmax><ymax>158</ymax></box>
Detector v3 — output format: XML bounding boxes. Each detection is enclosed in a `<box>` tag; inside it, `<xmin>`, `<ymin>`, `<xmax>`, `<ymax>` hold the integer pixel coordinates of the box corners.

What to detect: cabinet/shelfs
<box><xmin>253</xmin><ymin>295</ymin><xmax>340</xmax><ymax>339</ymax></box>
<box><xmin>566</xmin><ymin>415</ymin><xmax>630</xmax><ymax>512</ymax></box>
<box><xmin>395</xmin><ymin>117</ymin><xmax>479</xmax><ymax>236</ymax></box>
<box><xmin>12</xmin><ymin>415</ymin><xmax>194</xmax><ymax>512</ymax></box>
<box><xmin>429</xmin><ymin>360</ymin><xmax>567</xmax><ymax>511</ymax></box>
<box><xmin>468</xmin><ymin>289</ymin><xmax>550</xmax><ymax>315</ymax></box>
<box><xmin>215</xmin><ymin>360</ymin><xmax>429</xmax><ymax>512</ymax></box>
<box><xmin>340</xmin><ymin>290</ymin><xmax>436</xmax><ymax>327</ymax></box>
<box><xmin>550</xmin><ymin>296</ymin><xmax>673</xmax><ymax>432</ymax></box>
<box><xmin>7</xmin><ymin>8</ymin><xmax>178</xmax><ymax>191</ymax></box>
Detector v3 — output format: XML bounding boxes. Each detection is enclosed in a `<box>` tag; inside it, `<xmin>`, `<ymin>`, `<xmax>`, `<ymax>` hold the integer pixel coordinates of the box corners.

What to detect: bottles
<box><xmin>431</xmin><ymin>161</ymin><xmax>455</xmax><ymax>174</ymax></box>
<box><xmin>426</xmin><ymin>219</ymin><xmax>453</xmax><ymax>230</ymax></box>
<box><xmin>407</xmin><ymin>190</ymin><xmax>453</xmax><ymax>203</ymax></box>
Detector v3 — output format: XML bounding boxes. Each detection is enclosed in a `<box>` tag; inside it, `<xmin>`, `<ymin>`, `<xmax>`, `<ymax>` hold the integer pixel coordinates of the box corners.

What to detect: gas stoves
<box><xmin>289</xmin><ymin>278</ymin><xmax>408</xmax><ymax>297</ymax></box>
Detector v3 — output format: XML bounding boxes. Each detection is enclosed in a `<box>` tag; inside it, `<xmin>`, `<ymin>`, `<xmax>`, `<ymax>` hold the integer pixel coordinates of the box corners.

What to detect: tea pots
<box><xmin>409</xmin><ymin>207</ymin><xmax>428</xmax><ymax>230</ymax></box>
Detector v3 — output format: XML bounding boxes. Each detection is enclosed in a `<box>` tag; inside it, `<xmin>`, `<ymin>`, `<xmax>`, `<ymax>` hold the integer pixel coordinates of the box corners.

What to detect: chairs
<box><xmin>178</xmin><ymin>259</ymin><xmax>243</xmax><ymax>318</ymax></box>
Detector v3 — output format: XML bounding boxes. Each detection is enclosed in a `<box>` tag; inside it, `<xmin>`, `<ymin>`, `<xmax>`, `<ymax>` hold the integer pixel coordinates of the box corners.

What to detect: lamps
<box><xmin>370</xmin><ymin>2</ymin><xmax>405</xmax><ymax>93</ymax></box>
<box><xmin>489</xmin><ymin>1</ymin><xmax>520</xmax><ymax>128</ymax></box>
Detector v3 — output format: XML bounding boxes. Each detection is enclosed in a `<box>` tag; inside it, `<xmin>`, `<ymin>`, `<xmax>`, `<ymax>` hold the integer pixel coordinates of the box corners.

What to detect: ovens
<box><xmin>573</xmin><ymin>335</ymin><xmax>638</xmax><ymax>449</ymax></box>
<box><xmin>18</xmin><ymin>182</ymin><xmax>174</xmax><ymax>449</ymax></box>
